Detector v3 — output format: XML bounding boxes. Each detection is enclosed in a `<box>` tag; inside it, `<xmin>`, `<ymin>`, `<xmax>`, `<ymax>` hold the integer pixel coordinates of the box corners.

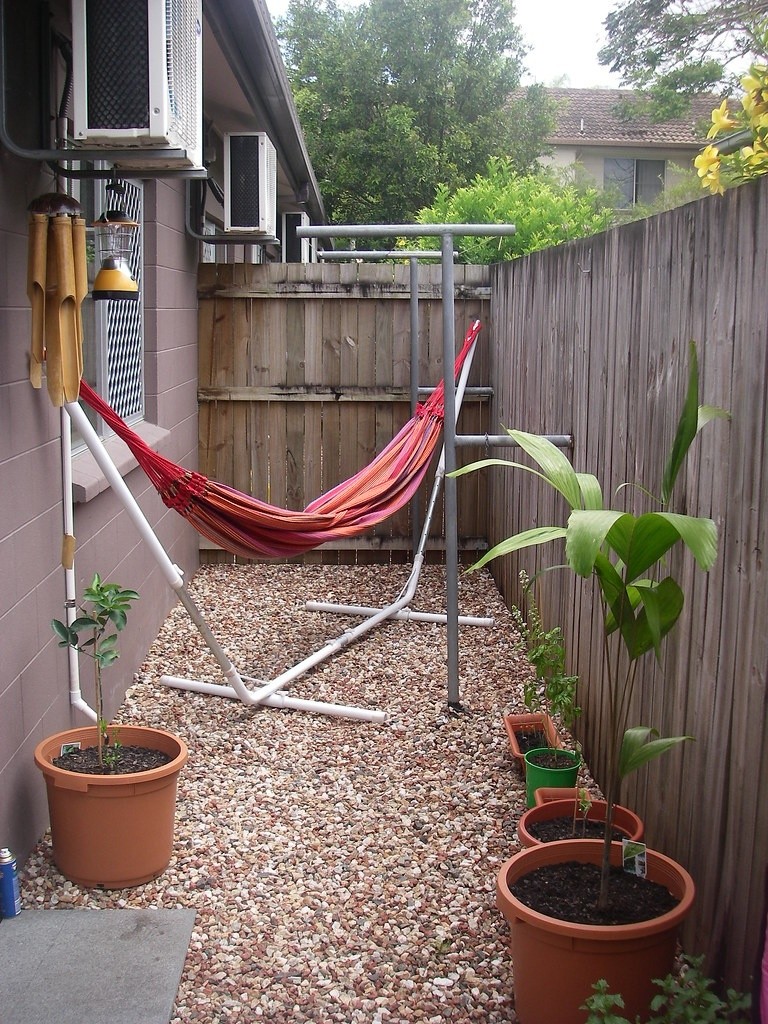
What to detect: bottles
<box><xmin>0</xmin><ymin>847</ymin><xmax>22</xmax><ymax>920</ymax></box>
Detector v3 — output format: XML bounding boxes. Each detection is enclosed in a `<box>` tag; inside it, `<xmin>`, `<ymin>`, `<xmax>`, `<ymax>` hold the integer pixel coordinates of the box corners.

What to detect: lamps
<box><xmin>88</xmin><ymin>166</ymin><xmax>139</xmax><ymax>298</ymax></box>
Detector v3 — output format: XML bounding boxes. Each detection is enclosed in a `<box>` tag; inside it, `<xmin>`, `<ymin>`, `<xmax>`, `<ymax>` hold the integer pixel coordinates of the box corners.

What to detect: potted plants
<box><xmin>443</xmin><ymin>331</ymin><xmax>732</xmax><ymax>1024</ymax></box>
<box><xmin>33</xmin><ymin>574</ymin><xmax>185</xmax><ymax>889</ymax></box>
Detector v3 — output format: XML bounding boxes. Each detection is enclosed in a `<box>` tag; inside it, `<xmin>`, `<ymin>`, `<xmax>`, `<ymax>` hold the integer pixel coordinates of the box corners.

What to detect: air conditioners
<box><xmin>70</xmin><ymin>1</ymin><xmax>204</xmax><ymax>165</ymax></box>
<box><xmin>221</xmin><ymin>132</ymin><xmax>326</xmax><ymax>264</ymax></box>
